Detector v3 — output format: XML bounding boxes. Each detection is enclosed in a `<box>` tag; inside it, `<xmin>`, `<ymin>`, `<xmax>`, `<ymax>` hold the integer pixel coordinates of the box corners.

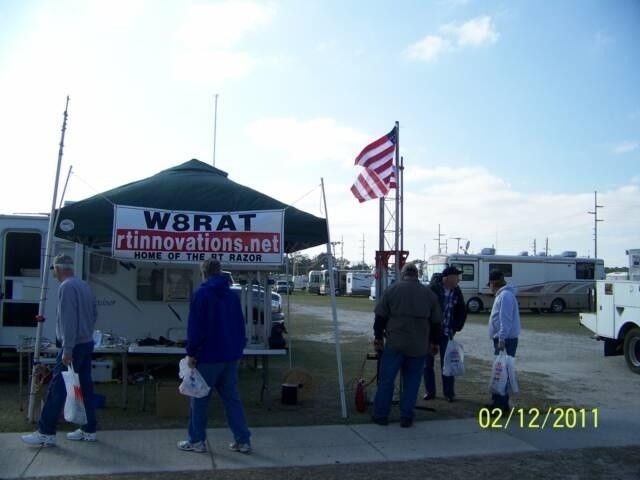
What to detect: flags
<box><xmin>350</xmin><ymin>125</ymin><xmax>398</xmax><ymax>203</ymax></box>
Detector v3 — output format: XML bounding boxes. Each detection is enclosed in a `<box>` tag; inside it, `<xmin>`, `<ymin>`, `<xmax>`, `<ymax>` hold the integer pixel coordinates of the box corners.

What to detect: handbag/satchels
<box><xmin>488</xmin><ymin>350</ymin><xmax>507</xmax><ymax>396</ymax></box>
<box><xmin>443</xmin><ymin>338</ymin><xmax>465</xmax><ymax>377</ymax></box>
<box><xmin>504</xmin><ymin>348</ymin><xmax>520</xmax><ymax>394</ymax></box>
<box><xmin>61</xmin><ymin>363</ymin><xmax>88</xmax><ymax>425</ymax></box>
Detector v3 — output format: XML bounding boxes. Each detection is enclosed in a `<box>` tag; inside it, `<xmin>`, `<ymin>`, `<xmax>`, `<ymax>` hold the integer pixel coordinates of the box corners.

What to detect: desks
<box><xmin>16</xmin><ymin>342</ymin><xmax>128</xmax><ymax>411</ymax></box>
<box><xmin>128</xmin><ymin>343</ymin><xmax>287</xmax><ymax>412</ymax></box>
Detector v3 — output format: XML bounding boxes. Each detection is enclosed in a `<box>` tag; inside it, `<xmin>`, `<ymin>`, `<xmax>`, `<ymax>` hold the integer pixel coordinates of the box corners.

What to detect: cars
<box><xmin>273</xmin><ymin>280</ymin><xmax>294</xmax><ymax>294</ymax></box>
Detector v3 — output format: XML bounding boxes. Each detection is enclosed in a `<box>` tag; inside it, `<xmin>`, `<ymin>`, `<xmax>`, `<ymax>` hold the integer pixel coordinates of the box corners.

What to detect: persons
<box><xmin>20</xmin><ymin>255</ymin><xmax>100</xmax><ymax>446</ymax></box>
<box><xmin>176</xmin><ymin>259</ymin><xmax>251</xmax><ymax>453</ymax></box>
<box><xmin>422</xmin><ymin>266</ymin><xmax>468</xmax><ymax>401</ymax></box>
<box><xmin>371</xmin><ymin>262</ymin><xmax>440</xmax><ymax>428</ymax></box>
<box><xmin>486</xmin><ymin>270</ymin><xmax>521</xmax><ymax>418</ymax></box>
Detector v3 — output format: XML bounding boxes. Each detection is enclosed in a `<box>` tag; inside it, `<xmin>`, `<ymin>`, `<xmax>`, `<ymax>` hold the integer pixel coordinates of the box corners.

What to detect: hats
<box><xmin>489</xmin><ymin>269</ymin><xmax>505</xmax><ymax>280</ymax></box>
<box><xmin>443</xmin><ymin>266</ymin><xmax>463</xmax><ymax>277</ymax></box>
<box><xmin>50</xmin><ymin>256</ymin><xmax>73</xmax><ymax>269</ymax></box>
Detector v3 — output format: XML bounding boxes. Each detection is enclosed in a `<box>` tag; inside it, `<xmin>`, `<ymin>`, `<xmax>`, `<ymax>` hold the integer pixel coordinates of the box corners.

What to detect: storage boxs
<box><xmin>155</xmin><ymin>381</ymin><xmax>191</xmax><ymax>417</ymax></box>
<box><xmin>90</xmin><ymin>360</ymin><xmax>112</xmax><ymax>383</ymax></box>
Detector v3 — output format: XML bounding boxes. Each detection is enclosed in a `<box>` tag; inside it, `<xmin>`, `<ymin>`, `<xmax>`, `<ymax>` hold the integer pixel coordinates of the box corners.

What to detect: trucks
<box><xmin>427</xmin><ymin>255</ymin><xmax>605</xmax><ymax>313</ymax></box>
<box><xmin>575</xmin><ymin>280</ymin><xmax>639</xmax><ymax>372</ymax></box>
<box><xmin>308</xmin><ymin>264</ymin><xmax>425</xmax><ymax>302</ymax></box>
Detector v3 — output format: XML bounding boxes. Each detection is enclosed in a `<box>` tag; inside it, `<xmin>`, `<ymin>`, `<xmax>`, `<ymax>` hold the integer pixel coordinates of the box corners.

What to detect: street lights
<box><xmin>330</xmin><ymin>242</ymin><xmax>340</xmax><ymax>267</ymax></box>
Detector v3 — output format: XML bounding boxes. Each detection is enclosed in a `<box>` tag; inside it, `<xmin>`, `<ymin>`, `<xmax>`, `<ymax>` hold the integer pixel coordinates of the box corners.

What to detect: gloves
<box><xmin>374</xmin><ymin>338</ymin><xmax>384</xmax><ymax>353</ymax></box>
<box><xmin>429</xmin><ymin>343</ymin><xmax>438</xmax><ymax>355</ymax></box>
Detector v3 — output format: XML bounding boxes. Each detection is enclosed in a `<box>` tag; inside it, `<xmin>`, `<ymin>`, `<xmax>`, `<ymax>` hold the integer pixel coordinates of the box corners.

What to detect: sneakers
<box><xmin>484</xmin><ymin>405</ymin><xmax>510</xmax><ymax>417</ymax></box>
<box><xmin>424</xmin><ymin>393</ymin><xmax>436</xmax><ymax>400</ymax></box>
<box><xmin>400</xmin><ymin>417</ymin><xmax>413</xmax><ymax>427</ymax></box>
<box><xmin>371</xmin><ymin>415</ymin><xmax>389</xmax><ymax>425</ymax></box>
<box><xmin>66</xmin><ymin>429</ymin><xmax>97</xmax><ymax>441</ymax></box>
<box><xmin>229</xmin><ymin>441</ymin><xmax>251</xmax><ymax>454</ymax></box>
<box><xmin>444</xmin><ymin>392</ymin><xmax>455</xmax><ymax>401</ymax></box>
<box><xmin>178</xmin><ymin>440</ymin><xmax>207</xmax><ymax>452</ymax></box>
<box><xmin>22</xmin><ymin>431</ymin><xmax>56</xmax><ymax>447</ymax></box>
